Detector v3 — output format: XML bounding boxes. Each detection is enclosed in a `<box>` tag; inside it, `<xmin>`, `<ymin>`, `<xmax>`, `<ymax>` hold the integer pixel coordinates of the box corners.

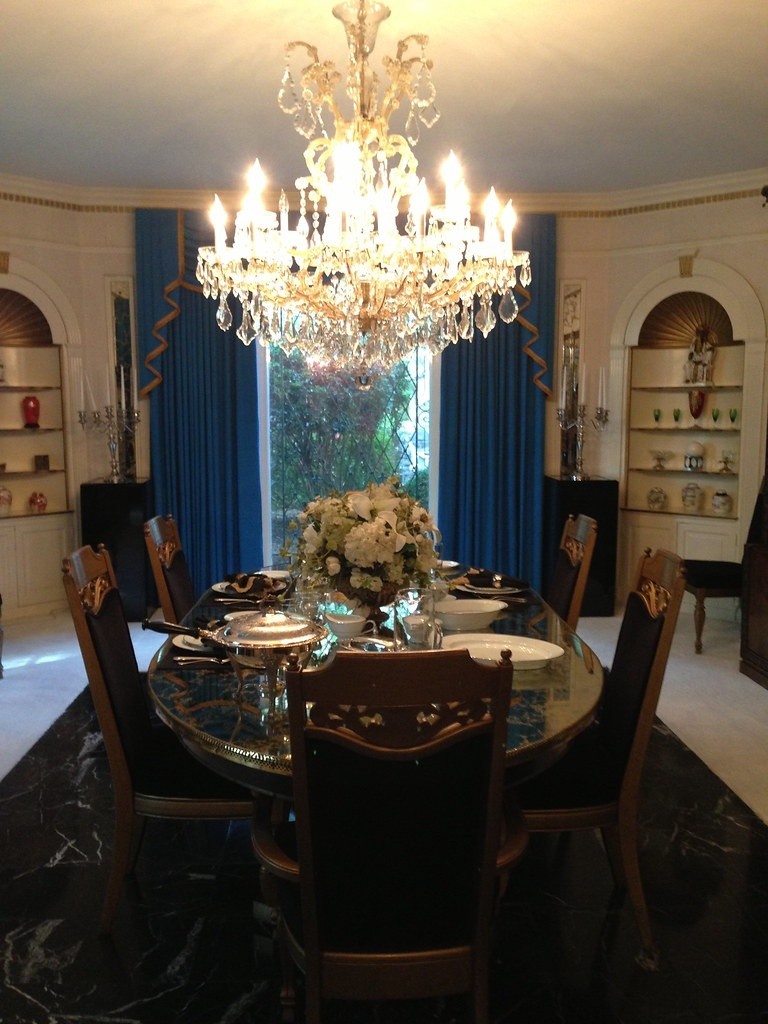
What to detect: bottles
<box><xmin>29</xmin><ymin>490</ymin><xmax>48</xmax><ymax>512</ymax></box>
<box><xmin>647</xmin><ymin>487</ymin><xmax>666</xmax><ymax>510</ymax></box>
<box><xmin>682</xmin><ymin>483</ymin><xmax>704</xmax><ymax>512</ymax></box>
<box><xmin>23</xmin><ymin>396</ymin><xmax>40</xmax><ymax>429</ymax></box>
<box><xmin>711</xmin><ymin>489</ymin><xmax>732</xmax><ymax>514</ymax></box>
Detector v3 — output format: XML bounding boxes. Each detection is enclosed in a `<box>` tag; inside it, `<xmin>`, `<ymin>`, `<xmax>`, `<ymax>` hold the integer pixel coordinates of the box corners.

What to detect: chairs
<box><xmin>547</xmin><ymin>514</ymin><xmax>598</xmax><ymax>632</ymax></box>
<box><xmin>143</xmin><ymin>514</ymin><xmax>196</xmax><ymax>637</ymax></box>
<box><xmin>682</xmin><ymin>473</ymin><xmax>768</xmax><ymax>655</ymax></box>
<box><xmin>282</xmin><ymin>647</ymin><xmax>513</xmax><ymax>1024</ymax></box>
<box><xmin>61</xmin><ymin>543</ymin><xmax>270</xmax><ymax>941</ymax></box>
<box><xmin>517</xmin><ymin>547</ymin><xmax>673</xmax><ymax>977</ymax></box>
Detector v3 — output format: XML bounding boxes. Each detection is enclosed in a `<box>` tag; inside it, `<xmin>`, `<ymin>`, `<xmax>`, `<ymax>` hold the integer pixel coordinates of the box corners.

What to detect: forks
<box><xmin>476</xmin><ymin>595</ymin><xmax>526</xmax><ymax>600</ymax></box>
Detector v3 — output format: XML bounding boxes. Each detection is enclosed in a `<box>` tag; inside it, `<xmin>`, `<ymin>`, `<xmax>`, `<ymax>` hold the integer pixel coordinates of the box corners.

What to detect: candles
<box><xmin>559</xmin><ymin>363</ymin><xmax>606</xmax><ymax>409</ymax></box>
<box><xmin>80</xmin><ymin>365</ymin><xmax>137</xmax><ymax>411</ymax></box>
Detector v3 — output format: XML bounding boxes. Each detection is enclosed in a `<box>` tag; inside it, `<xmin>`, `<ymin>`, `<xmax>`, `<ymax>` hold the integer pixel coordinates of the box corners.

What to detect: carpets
<box><xmin>0</xmin><ymin>671</ymin><xmax>768</xmax><ymax>1024</ymax></box>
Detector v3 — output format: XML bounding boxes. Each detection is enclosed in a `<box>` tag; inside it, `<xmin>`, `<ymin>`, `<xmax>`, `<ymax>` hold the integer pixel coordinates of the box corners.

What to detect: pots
<box><xmin>142</xmin><ymin>611</ymin><xmax>328</xmax><ymax>668</ymax></box>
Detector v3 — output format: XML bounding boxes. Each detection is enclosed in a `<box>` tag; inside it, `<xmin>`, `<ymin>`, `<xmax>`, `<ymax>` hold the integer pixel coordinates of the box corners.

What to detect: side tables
<box><xmin>546</xmin><ymin>473</ymin><xmax>619</xmax><ymax>617</ymax></box>
<box><xmin>80</xmin><ymin>477</ymin><xmax>159</xmax><ymax>622</ymax></box>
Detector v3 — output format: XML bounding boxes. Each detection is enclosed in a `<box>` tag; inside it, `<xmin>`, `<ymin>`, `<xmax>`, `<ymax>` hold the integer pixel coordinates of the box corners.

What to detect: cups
<box><xmin>34</xmin><ymin>454</ymin><xmax>49</xmax><ymax>470</ymax></box>
<box><xmin>394</xmin><ymin>588</ymin><xmax>437</xmax><ymax>650</ymax></box>
<box><xmin>290</xmin><ymin>592</ymin><xmax>326</xmax><ymax>627</ymax></box>
<box><xmin>401</xmin><ymin>615</ymin><xmax>431</xmax><ymax>643</ymax></box>
<box><xmin>320</xmin><ymin>610</ymin><xmax>375</xmax><ymax>640</ymax></box>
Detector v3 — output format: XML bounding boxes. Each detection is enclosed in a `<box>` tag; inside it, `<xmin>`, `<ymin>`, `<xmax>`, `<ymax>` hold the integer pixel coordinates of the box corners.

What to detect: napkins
<box><xmin>224</xmin><ymin>571</ymin><xmax>282</xmax><ymax>595</ymax></box>
<box><xmin>447</xmin><ymin>567</ymin><xmax>529</xmax><ymax>594</ymax></box>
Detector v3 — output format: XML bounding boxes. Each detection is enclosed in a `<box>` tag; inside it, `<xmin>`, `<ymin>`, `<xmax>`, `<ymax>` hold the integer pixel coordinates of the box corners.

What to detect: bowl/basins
<box><xmin>424</xmin><ymin>600</ymin><xmax>508</xmax><ymax>630</ymax></box>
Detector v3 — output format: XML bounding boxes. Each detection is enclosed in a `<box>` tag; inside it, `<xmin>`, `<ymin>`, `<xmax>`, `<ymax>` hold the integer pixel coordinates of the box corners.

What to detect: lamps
<box><xmin>195</xmin><ymin>0</ymin><xmax>532</xmax><ymax>391</ymax></box>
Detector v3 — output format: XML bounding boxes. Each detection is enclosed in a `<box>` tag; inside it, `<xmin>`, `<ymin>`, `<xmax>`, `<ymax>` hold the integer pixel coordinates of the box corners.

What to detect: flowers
<box><xmin>280</xmin><ymin>473</ymin><xmax>443</xmax><ymax>604</ymax></box>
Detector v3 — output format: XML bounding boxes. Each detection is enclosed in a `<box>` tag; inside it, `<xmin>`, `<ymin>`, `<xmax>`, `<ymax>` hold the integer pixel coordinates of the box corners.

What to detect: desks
<box><xmin>146</xmin><ymin>577</ymin><xmax>604</xmax><ymax>804</ymax></box>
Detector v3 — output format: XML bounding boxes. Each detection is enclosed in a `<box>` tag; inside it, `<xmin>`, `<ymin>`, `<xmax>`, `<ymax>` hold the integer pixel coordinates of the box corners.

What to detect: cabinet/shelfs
<box><xmin>0</xmin><ymin>344</ymin><xmax>82</xmax><ymax>621</ymax></box>
<box><xmin>604</xmin><ymin>339</ymin><xmax>762</xmax><ymax>625</ymax></box>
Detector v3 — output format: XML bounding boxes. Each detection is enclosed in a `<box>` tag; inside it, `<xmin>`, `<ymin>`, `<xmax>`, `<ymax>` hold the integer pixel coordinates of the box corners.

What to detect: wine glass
<box><xmin>654</xmin><ymin>409</ymin><xmax>660</xmax><ymax>429</ymax></box>
<box><xmin>712</xmin><ymin>408</ymin><xmax>719</xmax><ymax>428</ymax></box>
<box><xmin>673</xmin><ymin>408</ymin><xmax>681</xmax><ymax>428</ymax></box>
<box><xmin>729</xmin><ymin>408</ymin><xmax>736</xmax><ymax>429</ymax></box>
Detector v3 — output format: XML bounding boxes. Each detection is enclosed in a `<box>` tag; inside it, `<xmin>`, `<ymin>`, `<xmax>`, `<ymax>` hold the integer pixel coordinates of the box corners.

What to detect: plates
<box><xmin>433</xmin><ymin>633</ymin><xmax>565</xmax><ymax>669</ymax></box>
<box><xmin>172</xmin><ymin>634</ymin><xmax>207</xmax><ymax>651</ymax></box>
<box><xmin>254</xmin><ymin>570</ymin><xmax>295</xmax><ymax>579</ymax></box>
<box><xmin>335</xmin><ymin>636</ymin><xmax>404</xmax><ymax>652</ymax></box>
<box><xmin>455</xmin><ymin>583</ymin><xmax>521</xmax><ymax>594</ymax></box>
<box><xmin>437</xmin><ymin>559</ymin><xmax>458</xmax><ymax>568</ymax></box>
<box><xmin>212</xmin><ymin>582</ymin><xmax>230</xmax><ymax>593</ymax></box>
